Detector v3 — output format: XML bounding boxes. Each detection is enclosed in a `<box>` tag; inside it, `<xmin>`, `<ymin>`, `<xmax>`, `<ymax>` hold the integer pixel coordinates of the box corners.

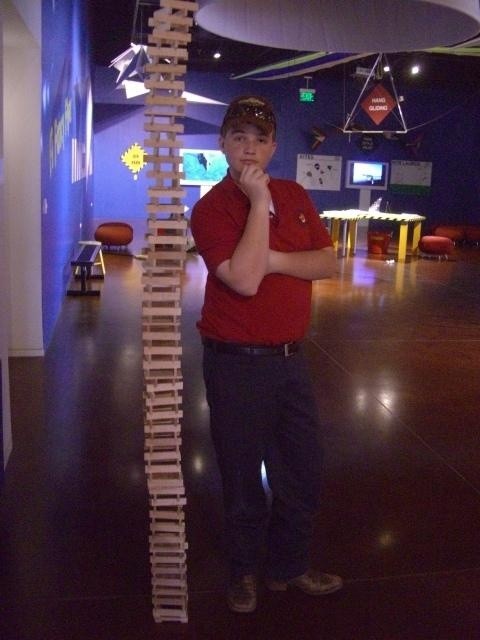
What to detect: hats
<box><xmin>224</xmin><ymin>96</ymin><xmax>274</xmax><ymax>137</ymax></box>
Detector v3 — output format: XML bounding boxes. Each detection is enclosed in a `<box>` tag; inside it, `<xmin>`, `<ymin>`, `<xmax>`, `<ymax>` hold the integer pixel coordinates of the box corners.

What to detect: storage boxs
<box><xmin>368</xmin><ymin>229</ymin><xmax>392</xmax><ymax>254</ymax></box>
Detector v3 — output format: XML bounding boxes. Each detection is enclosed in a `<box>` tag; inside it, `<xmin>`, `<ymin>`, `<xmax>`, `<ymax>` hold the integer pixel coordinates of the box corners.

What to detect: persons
<box><xmin>187</xmin><ymin>93</ymin><xmax>344</xmax><ymax>617</ymax></box>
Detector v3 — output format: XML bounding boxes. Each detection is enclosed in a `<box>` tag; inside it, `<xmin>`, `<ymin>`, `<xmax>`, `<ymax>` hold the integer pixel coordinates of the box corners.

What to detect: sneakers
<box><xmin>267</xmin><ymin>568</ymin><xmax>342</xmax><ymax>595</ymax></box>
<box><xmin>226</xmin><ymin>574</ymin><xmax>257</xmax><ymax>612</ymax></box>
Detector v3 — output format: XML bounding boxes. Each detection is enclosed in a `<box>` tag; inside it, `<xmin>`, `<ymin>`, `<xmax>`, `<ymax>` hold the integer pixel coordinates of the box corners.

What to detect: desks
<box><xmin>319</xmin><ymin>208</ymin><xmax>425</xmax><ymax>259</ymax></box>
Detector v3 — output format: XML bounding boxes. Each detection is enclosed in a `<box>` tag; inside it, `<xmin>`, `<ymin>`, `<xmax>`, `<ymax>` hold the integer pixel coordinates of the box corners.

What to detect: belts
<box><xmin>201</xmin><ymin>338</ymin><xmax>307</xmax><ymax>357</ymax></box>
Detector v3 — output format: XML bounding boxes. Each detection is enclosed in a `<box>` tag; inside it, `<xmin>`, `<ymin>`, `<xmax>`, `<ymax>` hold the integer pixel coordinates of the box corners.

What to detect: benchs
<box><xmin>66</xmin><ymin>241</ymin><xmax>102</xmax><ymax>295</ymax></box>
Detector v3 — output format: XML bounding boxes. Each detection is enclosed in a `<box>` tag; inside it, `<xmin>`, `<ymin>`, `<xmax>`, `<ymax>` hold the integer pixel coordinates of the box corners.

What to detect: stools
<box><xmin>418</xmin><ymin>236</ymin><xmax>456</xmax><ymax>256</ymax></box>
<box><xmin>433</xmin><ymin>223</ymin><xmax>480</xmax><ymax>244</ymax></box>
<box><xmin>94</xmin><ymin>222</ymin><xmax>133</xmax><ymax>249</ymax></box>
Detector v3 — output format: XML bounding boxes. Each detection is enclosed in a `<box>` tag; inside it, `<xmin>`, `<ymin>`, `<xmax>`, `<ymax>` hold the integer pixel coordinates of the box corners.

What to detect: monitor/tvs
<box><xmin>179</xmin><ymin>148</ymin><xmax>230</xmax><ymax>186</ymax></box>
<box><xmin>345</xmin><ymin>160</ymin><xmax>389</xmax><ymax>191</ymax></box>
